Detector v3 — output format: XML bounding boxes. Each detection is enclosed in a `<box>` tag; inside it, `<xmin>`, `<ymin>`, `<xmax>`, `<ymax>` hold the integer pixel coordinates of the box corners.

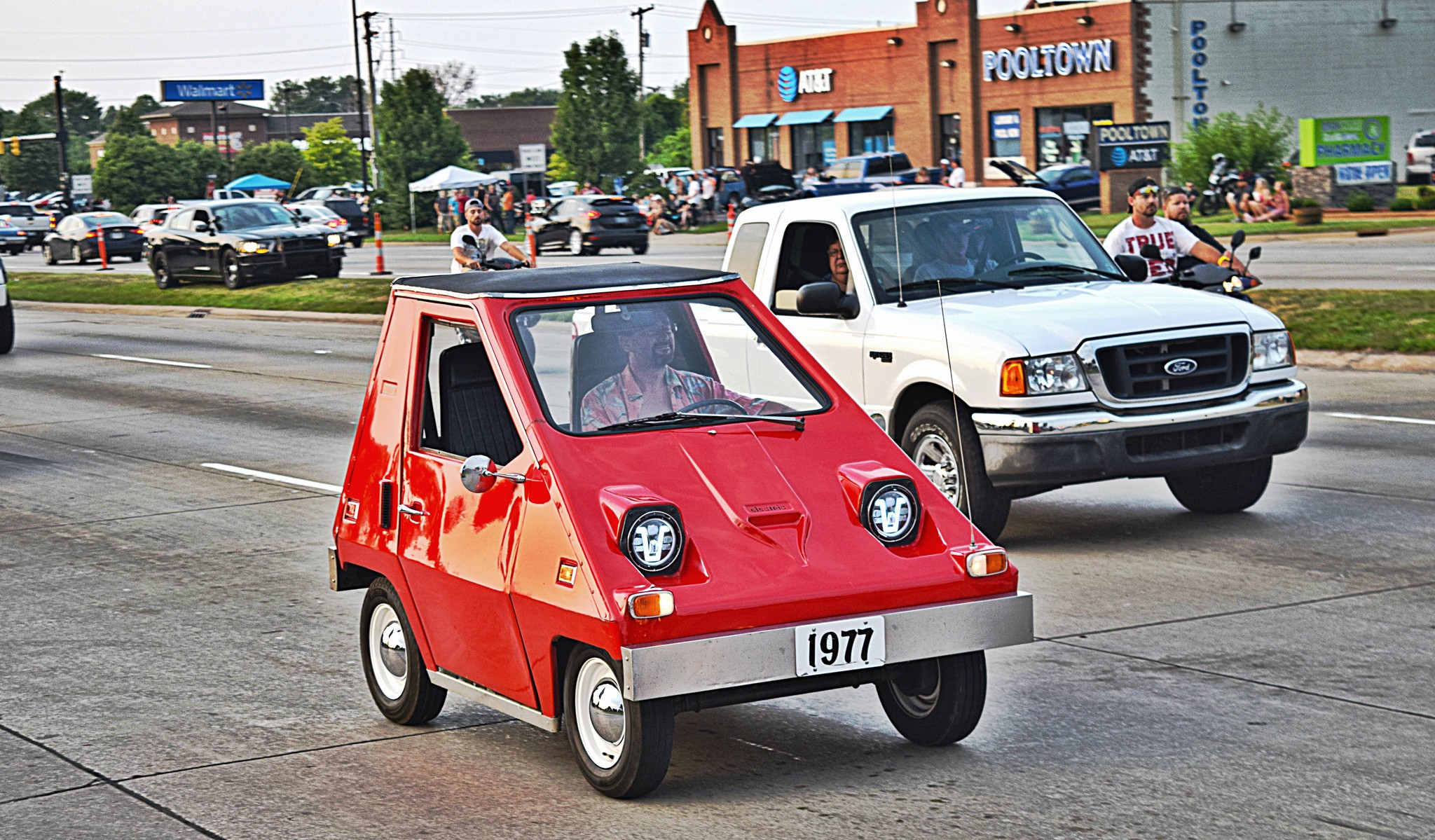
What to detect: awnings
<box><xmin>831</xmin><ymin>105</ymin><xmax>893</xmax><ymax>123</ymax></box>
<box><xmin>732</xmin><ymin>114</ymin><xmax>778</xmax><ymax>129</ymax></box>
<box><xmin>775</xmin><ymin>109</ymin><xmax>833</xmax><ymax>126</ymax></box>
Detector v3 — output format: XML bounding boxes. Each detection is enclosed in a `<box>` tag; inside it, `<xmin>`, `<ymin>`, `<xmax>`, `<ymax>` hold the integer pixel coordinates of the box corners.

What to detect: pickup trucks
<box><xmin>574</xmin><ymin>185</ymin><xmax>1309</xmax><ymax>544</ymax></box>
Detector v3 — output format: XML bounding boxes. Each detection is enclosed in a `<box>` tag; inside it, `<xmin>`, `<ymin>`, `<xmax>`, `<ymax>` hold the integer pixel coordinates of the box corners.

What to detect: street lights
<box><xmin>217</xmin><ymin>103</ymin><xmax>234</xmax><ymax>183</ymax></box>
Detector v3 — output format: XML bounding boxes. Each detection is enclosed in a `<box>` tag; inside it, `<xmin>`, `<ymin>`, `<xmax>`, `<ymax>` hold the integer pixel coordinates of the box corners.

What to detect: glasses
<box><xmin>1133</xmin><ymin>185</ymin><xmax>1159</xmax><ymax>197</ymax></box>
<box><xmin>634</xmin><ymin>323</ymin><xmax>678</xmax><ymax>335</ymax></box>
<box><xmin>827</xmin><ymin>248</ymin><xmax>842</xmax><ymax>256</ymax></box>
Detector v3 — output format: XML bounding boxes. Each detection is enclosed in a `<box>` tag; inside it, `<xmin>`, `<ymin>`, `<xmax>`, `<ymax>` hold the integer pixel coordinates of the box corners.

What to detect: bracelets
<box><xmin>1217</xmin><ymin>256</ymin><xmax>1231</xmax><ymax>266</ymax></box>
<box><xmin>780</xmin><ymin>406</ymin><xmax>789</xmax><ymax>413</ymax></box>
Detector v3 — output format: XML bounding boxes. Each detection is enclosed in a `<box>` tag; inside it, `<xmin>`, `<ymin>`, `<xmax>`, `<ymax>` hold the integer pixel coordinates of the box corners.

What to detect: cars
<box><xmin>333</xmin><ymin>262</ymin><xmax>1033</xmax><ymax>801</ymax></box>
<box><xmin>283</xmin><ymin>203</ymin><xmax>348</xmax><ymax>247</ymax></box>
<box><xmin>988</xmin><ymin>159</ymin><xmax>1101</xmax><ymax>213</ymax></box>
<box><xmin>462</xmin><ymin>150</ymin><xmax>948</xmax><ymax>272</ymax></box>
<box><xmin>0</xmin><ymin>257</ymin><xmax>15</xmax><ymax>355</ymax></box>
<box><xmin>149</xmin><ymin>199</ymin><xmax>348</xmax><ymax>291</ymax></box>
<box><xmin>0</xmin><ymin>189</ymin><xmax>355</xmax><ymax>266</ymax></box>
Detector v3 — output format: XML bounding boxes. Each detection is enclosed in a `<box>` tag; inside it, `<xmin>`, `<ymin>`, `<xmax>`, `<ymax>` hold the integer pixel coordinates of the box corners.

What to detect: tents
<box><xmin>409</xmin><ymin>164</ymin><xmax>498</xmax><ymax>233</ymax></box>
<box><xmin>223</xmin><ymin>174</ymin><xmax>291</xmax><ymax>200</ymax></box>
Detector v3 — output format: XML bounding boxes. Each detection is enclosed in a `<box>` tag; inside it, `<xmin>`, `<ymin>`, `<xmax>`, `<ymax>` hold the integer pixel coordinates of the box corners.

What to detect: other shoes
<box><xmin>1230</xmin><ymin>216</ymin><xmax>1242</xmax><ymax>222</ymax></box>
<box><xmin>1243</xmin><ymin>213</ymin><xmax>1255</xmax><ymax>224</ymax></box>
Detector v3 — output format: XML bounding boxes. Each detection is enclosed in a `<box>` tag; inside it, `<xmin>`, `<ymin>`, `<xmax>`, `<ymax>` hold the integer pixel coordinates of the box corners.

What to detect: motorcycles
<box><xmin>1197</xmin><ymin>164</ymin><xmax>1276</xmax><ymax>216</ymax></box>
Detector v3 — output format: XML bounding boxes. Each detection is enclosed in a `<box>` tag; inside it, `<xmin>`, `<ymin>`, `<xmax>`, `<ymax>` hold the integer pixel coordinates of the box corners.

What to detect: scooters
<box><xmin>1141</xmin><ymin>229</ymin><xmax>1262</xmax><ymax>303</ymax></box>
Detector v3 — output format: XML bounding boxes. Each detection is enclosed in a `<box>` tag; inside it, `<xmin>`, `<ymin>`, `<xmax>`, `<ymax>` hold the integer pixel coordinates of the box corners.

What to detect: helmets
<box><xmin>1211</xmin><ymin>152</ymin><xmax>1227</xmax><ymax>164</ymax></box>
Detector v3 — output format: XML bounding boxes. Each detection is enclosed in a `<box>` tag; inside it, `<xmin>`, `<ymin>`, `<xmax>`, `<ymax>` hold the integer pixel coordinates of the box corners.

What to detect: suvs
<box><xmin>293</xmin><ymin>199</ymin><xmax>370</xmax><ymax>248</ymax></box>
<box><xmin>1404</xmin><ymin>130</ymin><xmax>1435</xmax><ymax>185</ymax></box>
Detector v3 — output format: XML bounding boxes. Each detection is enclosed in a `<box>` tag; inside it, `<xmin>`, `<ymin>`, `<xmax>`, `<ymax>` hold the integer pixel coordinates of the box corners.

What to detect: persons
<box><xmin>433</xmin><ymin>180</ymin><xmax>536</xmax><ymax>234</ymax></box>
<box><xmin>913</xmin><ymin>213</ymin><xmax>999</xmax><ymax>283</ymax></box>
<box><xmin>1225</xmin><ymin>170</ymin><xmax>1290</xmax><ymax>223</ymax></box>
<box><xmin>581</xmin><ymin>310</ymin><xmax>799</xmax><ymax>435</ymax></box>
<box><xmin>1160</xmin><ymin>186</ymin><xmax>1252</xmax><ymax>277</ymax></box>
<box><xmin>939</xmin><ymin>157</ymin><xmax>966</xmax><ymax>188</ymax></box>
<box><xmin>820</xmin><ymin>234</ymin><xmax>896</xmax><ymax>295</ymax></box>
<box><xmin>1101</xmin><ymin>176</ymin><xmax>1244</xmax><ymax>290</ymax></box>
<box><xmin>915</xmin><ymin>167</ymin><xmax>931</xmax><ymax>184</ymax></box>
<box><xmin>578</xmin><ymin>180</ymin><xmax>604</xmax><ymax>196</ymax></box>
<box><xmin>633</xmin><ymin>165</ymin><xmax>722</xmax><ymax>235</ymax></box>
<box><xmin>450</xmin><ymin>198</ymin><xmax>532</xmax><ymax>274</ymax></box>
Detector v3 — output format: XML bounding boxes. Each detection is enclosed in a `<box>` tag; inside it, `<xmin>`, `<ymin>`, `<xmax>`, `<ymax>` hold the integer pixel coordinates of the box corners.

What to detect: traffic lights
<box><xmin>11</xmin><ymin>137</ymin><xmax>21</xmax><ymax>156</ymax></box>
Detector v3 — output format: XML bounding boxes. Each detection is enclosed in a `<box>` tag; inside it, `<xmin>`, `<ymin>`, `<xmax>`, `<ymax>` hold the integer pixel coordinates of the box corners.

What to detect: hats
<box><xmin>1127</xmin><ymin>177</ymin><xmax>1157</xmax><ymax>213</ymax></box>
<box><xmin>1236</xmin><ymin>177</ymin><xmax>1248</xmax><ymax>182</ymax></box>
<box><xmin>464</xmin><ymin>198</ymin><xmax>484</xmax><ymax>212</ymax></box>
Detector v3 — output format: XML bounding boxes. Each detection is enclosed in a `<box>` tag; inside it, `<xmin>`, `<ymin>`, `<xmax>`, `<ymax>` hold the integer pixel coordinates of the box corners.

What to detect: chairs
<box><xmin>785</xmin><ymin>227</ymin><xmax>833</xmax><ymax>290</ymax></box>
<box><xmin>566</xmin><ymin>329</ymin><xmax>631</xmax><ymax>434</ymax></box>
<box><xmin>437</xmin><ymin>340</ymin><xmax>528</xmax><ymax>468</ymax></box>
<box><xmin>895</xmin><ymin>220</ymin><xmax>941</xmax><ymax>287</ymax></box>
